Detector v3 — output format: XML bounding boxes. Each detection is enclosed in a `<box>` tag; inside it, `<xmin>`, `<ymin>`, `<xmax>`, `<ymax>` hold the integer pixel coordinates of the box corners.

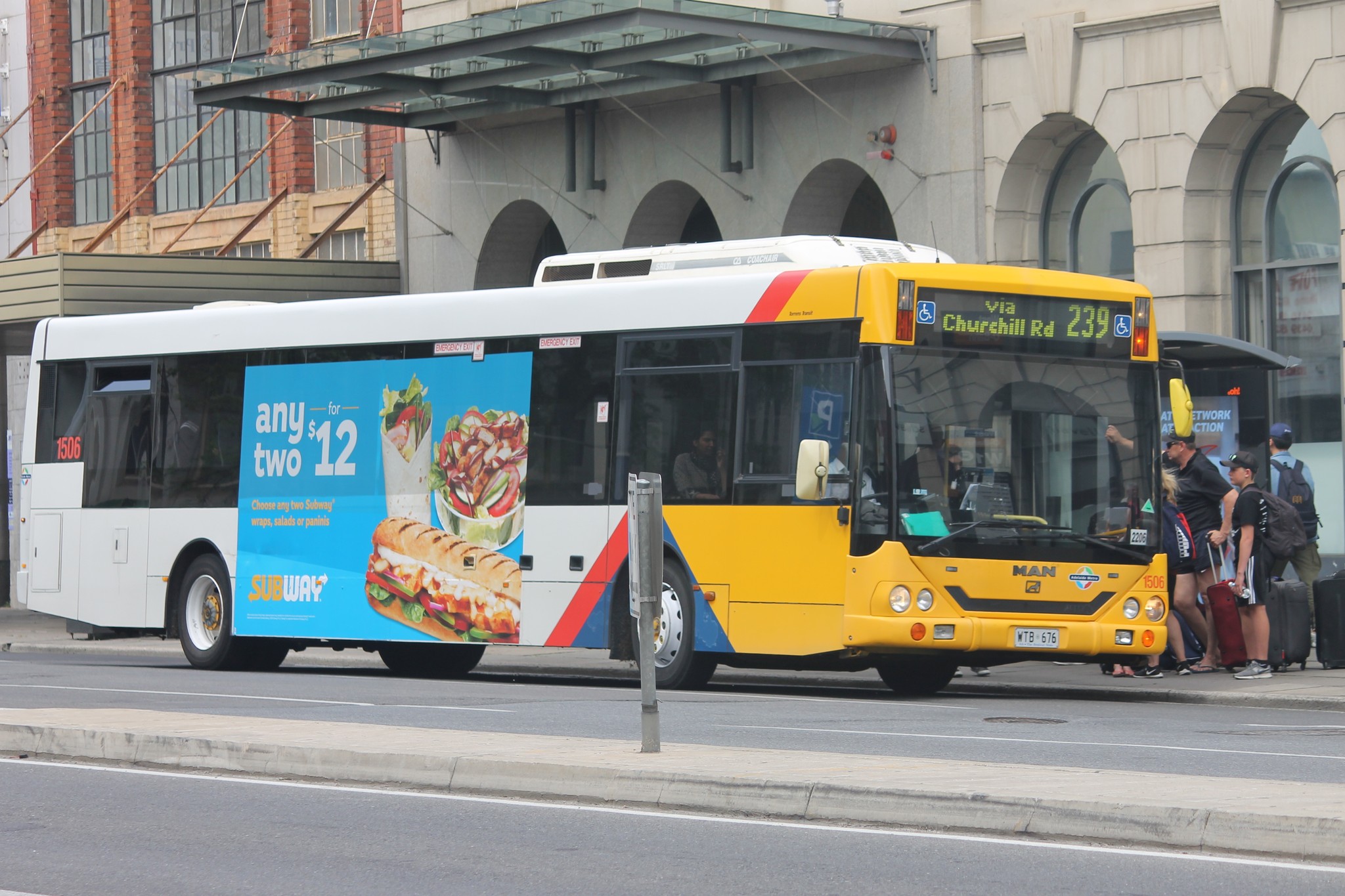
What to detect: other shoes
<box><xmin>975</xmin><ymin>666</ymin><xmax>991</xmax><ymax>676</ymax></box>
<box><xmin>953</xmin><ymin>669</ymin><xmax>963</xmax><ymax>678</ymax></box>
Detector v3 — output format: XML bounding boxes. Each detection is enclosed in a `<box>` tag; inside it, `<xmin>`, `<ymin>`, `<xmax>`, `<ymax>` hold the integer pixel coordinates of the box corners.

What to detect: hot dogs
<box><xmin>365</xmin><ymin>515</ymin><xmax>522</xmax><ymax>643</ymax></box>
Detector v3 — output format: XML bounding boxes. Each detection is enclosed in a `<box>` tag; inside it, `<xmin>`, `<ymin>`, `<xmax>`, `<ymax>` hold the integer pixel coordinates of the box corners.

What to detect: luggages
<box><xmin>1311</xmin><ymin>568</ymin><xmax>1345</xmax><ymax>670</ymax></box>
<box><xmin>1259</xmin><ymin>575</ymin><xmax>1312</xmax><ymax>672</ymax></box>
<box><xmin>1204</xmin><ymin>533</ymin><xmax>1248</xmax><ymax>670</ymax></box>
<box><xmin>1157</xmin><ymin>610</ymin><xmax>1206</xmax><ymax>670</ymax></box>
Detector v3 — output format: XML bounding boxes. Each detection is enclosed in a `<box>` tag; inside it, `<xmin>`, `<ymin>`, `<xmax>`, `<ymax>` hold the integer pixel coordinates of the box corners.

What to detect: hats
<box><xmin>1167</xmin><ymin>425</ymin><xmax>1195</xmax><ymax>443</ymax></box>
<box><xmin>1220</xmin><ymin>451</ymin><xmax>1260</xmax><ymax>469</ymax></box>
<box><xmin>1269</xmin><ymin>423</ymin><xmax>1293</xmax><ymax>439</ymax></box>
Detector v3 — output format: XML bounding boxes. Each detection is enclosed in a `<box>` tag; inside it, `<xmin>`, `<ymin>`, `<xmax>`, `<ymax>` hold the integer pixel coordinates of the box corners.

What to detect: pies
<box><xmin>379</xmin><ymin>373</ymin><xmax>433</xmax><ymax>528</ymax></box>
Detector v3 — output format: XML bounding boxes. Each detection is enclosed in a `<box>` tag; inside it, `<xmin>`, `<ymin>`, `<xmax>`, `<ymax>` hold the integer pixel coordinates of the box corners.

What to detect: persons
<box><xmin>900</xmin><ymin>425</ymin><xmax>966</xmax><ymax>520</ymax></box>
<box><xmin>1105</xmin><ymin>423</ymin><xmax>1323</xmax><ymax>680</ymax></box>
<box><xmin>825</xmin><ymin>441</ymin><xmax>880</xmax><ymax>510</ymax></box>
<box><xmin>673</xmin><ymin>425</ymin><xmax>726</xmax><ymax>501</ymax></box>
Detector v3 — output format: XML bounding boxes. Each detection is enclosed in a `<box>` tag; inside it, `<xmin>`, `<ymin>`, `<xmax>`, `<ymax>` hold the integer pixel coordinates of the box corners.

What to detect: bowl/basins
<box><xmin>434</xmin><ymin>488</ymin><xmax>526</xmax><ymax>552</ymax></box>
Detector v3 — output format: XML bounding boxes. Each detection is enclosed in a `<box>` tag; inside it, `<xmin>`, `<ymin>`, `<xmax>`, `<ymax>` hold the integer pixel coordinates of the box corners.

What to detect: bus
<box><xmin>15</xmin><ymin>234</ymin><xmax>1194</xmax><ymax>691</ymax></box>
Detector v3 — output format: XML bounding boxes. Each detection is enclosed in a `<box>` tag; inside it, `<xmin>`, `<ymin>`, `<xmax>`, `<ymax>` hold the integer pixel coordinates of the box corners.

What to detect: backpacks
<box><xmin>1269</xmin><ymin>459</ymin><xmax>1323</xmax><ymax>539</ymax></box>
<box><xmin>1240</xmin><ymin>485</ymin><xmax>1307</xmax><ymax>561</ymax></box>
<box><xmin>1158</xmin><ymin>492</ymin><xmax>1197</xmax><ymax>570</ymax></box>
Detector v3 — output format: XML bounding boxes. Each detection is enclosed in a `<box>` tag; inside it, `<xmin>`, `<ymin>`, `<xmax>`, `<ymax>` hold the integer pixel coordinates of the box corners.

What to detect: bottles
<box><xmin>1228</xmin><ymin>581</ymin><xmax>1252</xmax><ymax>599</ymax></box>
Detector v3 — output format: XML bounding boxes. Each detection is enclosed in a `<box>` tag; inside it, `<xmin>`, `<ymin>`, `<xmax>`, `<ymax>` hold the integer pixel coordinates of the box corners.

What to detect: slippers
<box><xmin>1112</xmin><ymin>670</ymin><xmax>1135</xmax><ymax>678</ymax></box>
<box><xmin>1188</xmin><ymin>661</ymin><xmax>1220</xmax><ymax>673</ymax></box>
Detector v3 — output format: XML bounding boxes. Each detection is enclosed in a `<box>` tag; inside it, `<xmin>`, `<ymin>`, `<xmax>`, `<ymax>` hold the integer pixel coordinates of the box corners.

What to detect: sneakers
<box><xmin>1132</xmin><ymin>665</ymin><xmax>1163</xmax><ymax>678</ymax></box>
<box><xmin>1232</xmin><ymin>660</ymin><xmax>1273</xmax><ymax>680</ymax></box>
<box><xmin>1175</xmin><ymin>661</ymin><xmax>1190</xmax><ymax>675</ymax></box>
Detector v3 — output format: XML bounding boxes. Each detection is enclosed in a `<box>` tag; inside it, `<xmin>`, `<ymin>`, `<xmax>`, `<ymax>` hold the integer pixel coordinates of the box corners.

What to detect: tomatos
<box><xmin>441</xmin><ymin>411</ymin><xmax>521</xmax><ymax>517</ymax></box>
